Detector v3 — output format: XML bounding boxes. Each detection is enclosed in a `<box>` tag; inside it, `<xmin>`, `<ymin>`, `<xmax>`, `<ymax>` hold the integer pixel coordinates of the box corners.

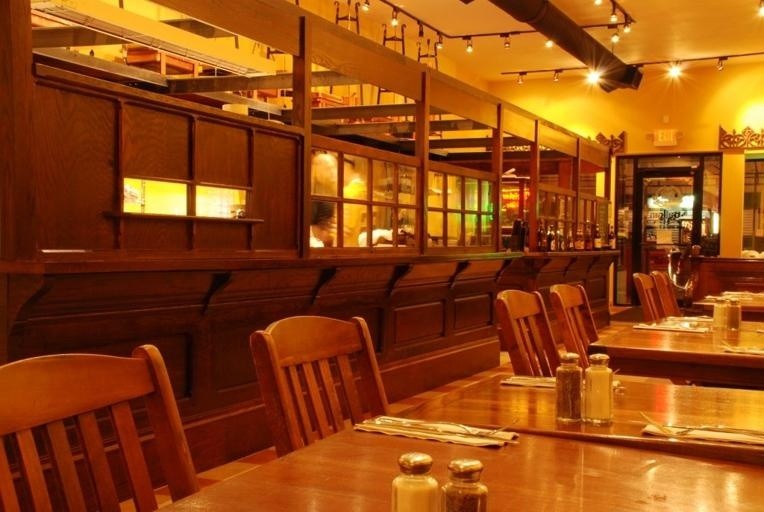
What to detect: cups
<box><xmin>692</xmin><ymin>245</ymin><xmax>701</xmax><ymax>256</ymax></box>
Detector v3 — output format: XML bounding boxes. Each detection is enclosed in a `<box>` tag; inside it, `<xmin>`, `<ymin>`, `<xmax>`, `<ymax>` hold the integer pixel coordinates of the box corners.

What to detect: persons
<box><xmin>310</xmin><ymin>153</ymin><xmax>370</xmax><ymax>248</ymax></box>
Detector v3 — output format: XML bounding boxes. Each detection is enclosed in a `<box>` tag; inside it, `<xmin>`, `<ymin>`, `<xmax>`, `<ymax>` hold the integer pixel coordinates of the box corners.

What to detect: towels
<box><xmin>631</xmin><ymin>321</ymin><xmax>712</xmax><ymax>335</ymax></box>
<box><xmin>498</xmin><ymin>374</ymin><xmax>622</xmax><ymax>392</ymax></box>
<box><xmin>640</xmin><ymin>417</ymin><xmax>764</xmax><ymax>446</ymax></box>
<box><xmin>350</xmin><ymin>411</ymin><xmax>517</xmax><ymax>449</ymax></box>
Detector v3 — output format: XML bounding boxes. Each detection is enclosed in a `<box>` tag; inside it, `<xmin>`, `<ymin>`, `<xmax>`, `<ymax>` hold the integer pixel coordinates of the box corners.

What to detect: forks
<box><xmin>377</xmin><ymin>417</ymin><xmax>519</xmax><ymax>437</ymax></box>
<box><xmin>637</xmin><ymin>410</ymin><xmax>764</xmax><ymax>438</ymax></box>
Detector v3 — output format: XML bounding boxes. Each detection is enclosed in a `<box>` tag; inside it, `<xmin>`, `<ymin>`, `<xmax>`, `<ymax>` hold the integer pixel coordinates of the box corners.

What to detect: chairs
<box><xmin>550</xmin><ymin>280</ymin><xmax>599</xmax><ymax>360</ymax></box>
<box><xmin>0</xmin><ymin>338</ymin><xmax>201</xmax><ymax>512</ymax></box>
<box><xmin>493</xmin><ymin>286</ymin><xmax>587</xmax><ymax>377</ymax></box>
<box><xmin>632</xmin><ymin>272</ymin><xmax>661</xmax><ymax>322</ymax></box>
<box><xmin>649</xmin><ymin>269</ymin><xmax>685</xmax><ymax>316</ymax></box>
<box><xmin>247</xmin><ymin>312</ymin><xmax>393</xmax><ymax>460</ymax></box>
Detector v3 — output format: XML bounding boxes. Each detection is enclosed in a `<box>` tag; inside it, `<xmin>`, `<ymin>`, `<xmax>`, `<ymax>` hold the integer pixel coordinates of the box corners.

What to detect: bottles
<box><xmin>537</xmin><ymin>224</ymin><xmax>616</xmax><ymax>253</ymax></box>
<box><xmin>392</xmin><ymin>453</ymin><xmax>438</xmax><ymax>511</ymax></box>
<box><xmin>441</xmin><ymin>459</ymin><xmax>489</xmax><ymax>512</ymax></box>
<box><xmin>713</xmin><ymin>297</ymin><xmax>727</xmax><ymax>327</ymax></box>
<box><xmin>581</xmin><ymin>352</ymin><xmax>614</xmax><ymax>424</ymax></box>
<box><xmin>554</xmin><ymin>352</ymin><xmax>582</xmax><ymax>423</ymax></box>
<box><xmin>728</xmin><ymin>297</ymin><xmax>742</xmax><ymax>331</ymax></box>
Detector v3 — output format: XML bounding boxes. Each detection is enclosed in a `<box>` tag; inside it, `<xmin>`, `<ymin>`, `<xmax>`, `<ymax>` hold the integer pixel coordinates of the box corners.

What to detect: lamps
<box><xmin>391</xmin><ymin>10</ymin><xmax>400</xmax><ymax>28</ymax></box>
<box><xmin>517</xmin><ymin>74</ymin><xmax>525</xmax><ymax>86</ymax></box>
<box><xmin>553</xmin><ymin>72</ymin><xmax>561</xmax><ymax>81</ymax></box>
<box><xmin>715</xmin><ymin>59</ymin><xmax>725</xmax><ymax>73</ymax></box>
<box><xmin>503</xmin><ymin>36</ymin><xmax>512</xmax><ymax>49</ymax></box>
<box><xmin>624</xmin><ymin>20</ymin><xmax>631</xmax><ymax>32</ymax></box>
<box><xmin>416</xmin><ymin>24</ymin><xmax>426</xmax><ymax>41</ymax></box>
<box><xmin>436</xmin><ymin>35</ymin><xmax>445</xmax><ymax>46</ymax></box>
<box><xmin>612</xmin><ymin>31</ymin><xmax>619</xmax><ymax>42</ymax></box>
<box><xmin>611</xmin><ymin>8</ymin><xmax>619</xmax><ymax>22</ymax></box>
<box><xmin>465</xmin><ymin>38</ymin><xmax>475</xmax><ymax>53</ymax></box>
<box><xmin>359</xmin><ymin>1</ymin><xmax>372</xmax><ymax>11</ymax></box>
